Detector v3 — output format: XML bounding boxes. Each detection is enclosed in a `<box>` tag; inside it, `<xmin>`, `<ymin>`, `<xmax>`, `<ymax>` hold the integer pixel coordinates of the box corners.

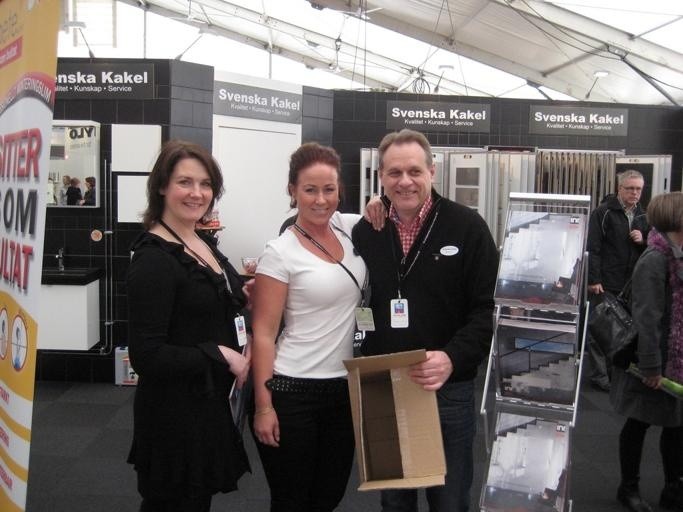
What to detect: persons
<box><xmin>47</xmin><ymin>178</ymin><xmax>56</xmax><ymax>205</ymax></box>
<box><xmin>240</xmin><ymin>128</ymin><xmax>502</xmax><ymax>512</ymax></box>
<box><xmin>126</xmin><ymin>136</ymin><xmax>255</xmax><ymax>512</ymax></box>
<box><xmin>610</xmin><ymin>191</ymin><xmax>683</xmax><ymax>512</ymax></box>
<box><xmin>586</xmin><ymin>169</ymin><xmax>654</xmax><ymax>392</ymax></box>
<box><xmin>79</xmin><ymin>176</ymin><xmax>96</xmax><ymax>205</ymax></box>
<box><xmin>247</xmin><ymin>142</ymin><xmax>389</xmax><ymax>512</ymax></box>
<box><xmin>60</xmin><ymin>175</ymin><xmax>71</xmax><ymax>205</ymax></box>
<box><xmin>66</xmin><ymin>177</ymin><xmax>83</xmax><ymax>205</ymax></box>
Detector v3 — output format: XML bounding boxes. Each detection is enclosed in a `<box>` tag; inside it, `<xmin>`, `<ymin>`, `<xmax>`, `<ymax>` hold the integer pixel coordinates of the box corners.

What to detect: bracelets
<box><xmin>254</xmin><ymin>404</ymin><xmax>274</xmax><ymax>418</ymax></box>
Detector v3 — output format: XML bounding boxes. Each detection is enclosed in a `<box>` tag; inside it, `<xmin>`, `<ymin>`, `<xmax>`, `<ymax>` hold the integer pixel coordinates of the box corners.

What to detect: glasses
<box><xmin>621</xmin><ymin>183</ymin><xmax>643</xmax><ymax>193</ymax></box>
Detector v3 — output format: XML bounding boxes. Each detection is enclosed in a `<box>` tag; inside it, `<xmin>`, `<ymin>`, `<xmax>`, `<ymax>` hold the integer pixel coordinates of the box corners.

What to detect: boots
<box><xmin>617</xmin><ymin>474</ymin><xmax>655</xmax><ymax>512</ymax></box>
<box><xmin>660</xmin><ymin>475</ymin><xmax>683</xmax><ymax>510</ymax></box>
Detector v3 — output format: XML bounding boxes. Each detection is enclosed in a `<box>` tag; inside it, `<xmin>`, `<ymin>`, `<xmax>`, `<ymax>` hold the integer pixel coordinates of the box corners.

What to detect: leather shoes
<box><xmin>590</xmin><ymin>380</ymin><xmax>611</xmax><ymax>392</ymax></box>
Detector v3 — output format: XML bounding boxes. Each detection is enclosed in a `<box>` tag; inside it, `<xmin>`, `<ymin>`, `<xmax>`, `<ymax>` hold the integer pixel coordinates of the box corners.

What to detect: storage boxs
<box><xmin>341</xmin><ymin>347</ymin><xmax>448</xmax><ymax>493</ymax></box>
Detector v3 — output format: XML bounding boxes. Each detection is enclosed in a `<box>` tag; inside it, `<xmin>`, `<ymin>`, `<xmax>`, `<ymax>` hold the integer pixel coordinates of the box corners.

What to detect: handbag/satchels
<box><xmin>588</xmin><ymin>297</ymin><xmax>639</xmax><ymax>370</ymax></box>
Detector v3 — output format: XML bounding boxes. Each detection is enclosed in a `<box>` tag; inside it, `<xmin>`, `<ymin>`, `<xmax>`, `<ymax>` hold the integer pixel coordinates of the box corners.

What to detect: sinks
<box><xmin>42</xmin><ymin>269</ymin><xmax>87</xmax><ymax>276</ymax></box>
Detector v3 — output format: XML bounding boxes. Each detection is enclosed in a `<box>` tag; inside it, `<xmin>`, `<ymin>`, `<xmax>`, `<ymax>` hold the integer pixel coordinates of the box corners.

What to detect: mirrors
<box><xmin>43</xmin><ymin>119</ymin><xmax>101</xmax><ymax>210</ymax></box>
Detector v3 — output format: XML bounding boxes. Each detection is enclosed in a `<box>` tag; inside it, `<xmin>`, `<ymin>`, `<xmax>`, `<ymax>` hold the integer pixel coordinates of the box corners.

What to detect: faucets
<box><xmin>55</xmin><ymin>248</ymin><xmax>66</xmax><ymax>269</ymax></box>
